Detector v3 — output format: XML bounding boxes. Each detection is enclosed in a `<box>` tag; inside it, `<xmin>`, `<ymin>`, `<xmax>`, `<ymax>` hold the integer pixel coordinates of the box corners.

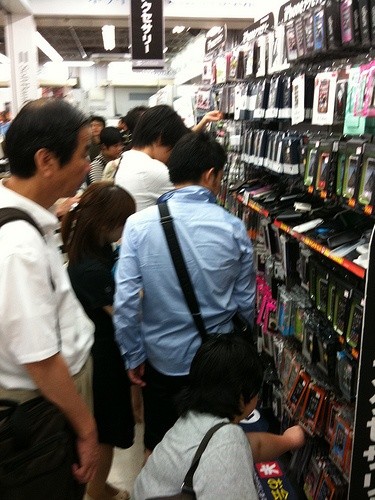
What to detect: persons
<box><xmin>1</xmin><ymin>103</ymin><xmax>225</xmax><ymax>426</ymax></box>
<box><xmin>111</xmin><ymin>131</ymin><xmax>257</xmax><ymax>467</ymax></box>
<box><xmin>60</xmin><ymin>180</ymin><xmax>144</xmax><ymax>500</ymax></box>
<box><xmin>127</xmin><ymin>331</ymin><xmax>306</xmax><ymax>500</ymax></box>
<box><xmin>0</xmin><ymin>96</ymin><xmax>102</xmax><ymax>500</ymax></box>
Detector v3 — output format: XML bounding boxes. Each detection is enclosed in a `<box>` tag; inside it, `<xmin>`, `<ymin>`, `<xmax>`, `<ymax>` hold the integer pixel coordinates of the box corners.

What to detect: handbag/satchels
<box><xmin>205</xmin><ymin>312</ymin><xmax>253</xmax><ymax>343</ymax></box>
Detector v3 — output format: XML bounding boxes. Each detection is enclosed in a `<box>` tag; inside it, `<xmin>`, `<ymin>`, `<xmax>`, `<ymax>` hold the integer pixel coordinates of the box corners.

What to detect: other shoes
<box><xmin>84</xmin><ymin>488</ymin><xmax>129</xmax><ymax>500</ymax></box>
